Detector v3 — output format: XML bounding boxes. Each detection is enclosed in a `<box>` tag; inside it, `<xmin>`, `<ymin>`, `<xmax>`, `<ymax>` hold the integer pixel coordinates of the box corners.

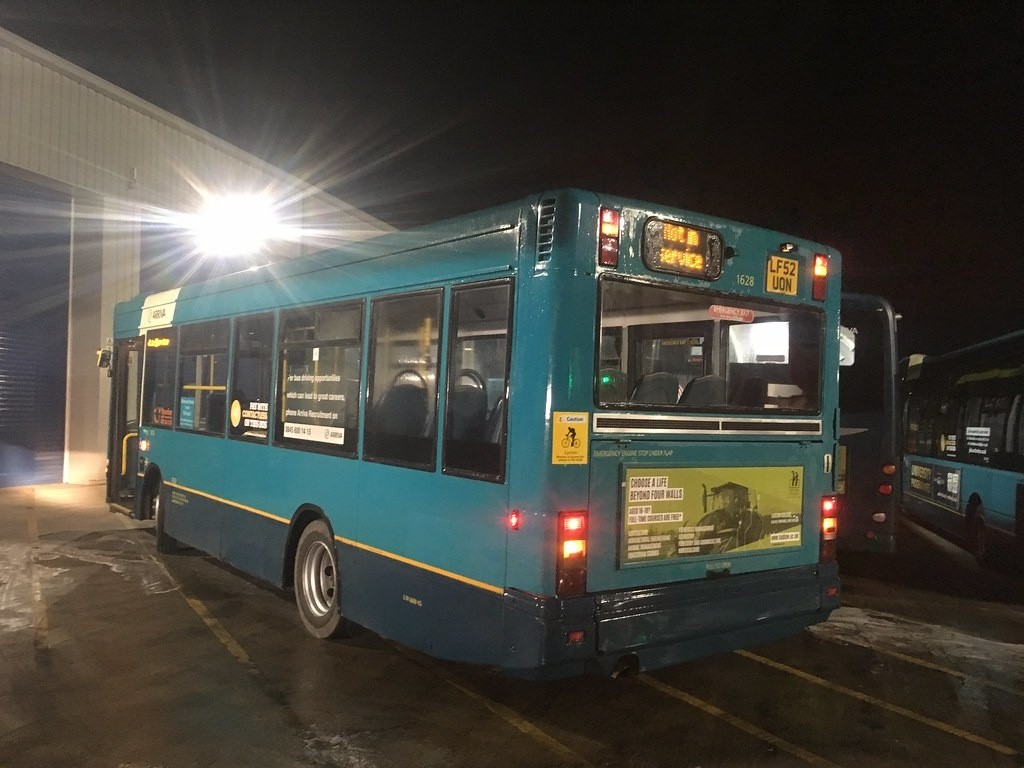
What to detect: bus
<box><xmin>96</xmin><ymin>188</ymin><xmax>844</xmax><ymax>684</ymax></box>
<box><xmin>877</xmin><ymin>329</ymin><xmax>1024</xmax><ymax>546</ymax></box>
<box><xmin>287</xmin><ymin>295</ymin><xmax>899</xmax><ymax>555</ymax></box>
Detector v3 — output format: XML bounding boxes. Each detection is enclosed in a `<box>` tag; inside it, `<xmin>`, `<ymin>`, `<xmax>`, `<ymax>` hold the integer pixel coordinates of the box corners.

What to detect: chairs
<box><xmin>205</xmin><ymin>368</ymin><xmax>503</xmax><ymax>444</ymax></box>
<box><xmin>598</xmin><ymin>368</ymin><xmax>768</xmax><ymax>408</ymax></box>
